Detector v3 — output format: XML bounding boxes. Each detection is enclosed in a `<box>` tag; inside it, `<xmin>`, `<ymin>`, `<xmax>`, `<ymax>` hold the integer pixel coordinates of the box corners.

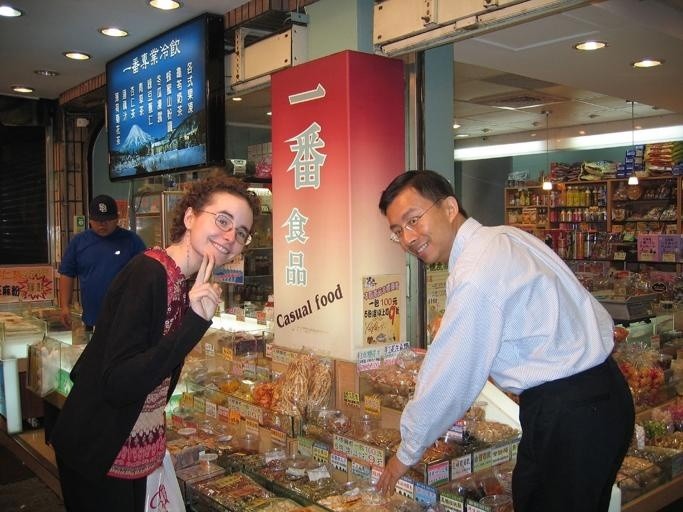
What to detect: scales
<box><xmin>593</xmin><ymin>295</ymin><xmax>658</xmax><ymax>327</ymax></box>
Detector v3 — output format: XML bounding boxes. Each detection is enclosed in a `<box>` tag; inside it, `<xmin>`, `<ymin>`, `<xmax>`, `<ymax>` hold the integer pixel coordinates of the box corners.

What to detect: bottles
<box><xmin>504</xmin><ymin>185</ymin><xmax>609</xmax><ymax>263</ymax></box>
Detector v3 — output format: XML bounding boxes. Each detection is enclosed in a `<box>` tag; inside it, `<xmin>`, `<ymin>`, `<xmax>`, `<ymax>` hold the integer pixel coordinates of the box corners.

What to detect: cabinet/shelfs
<box><xmin>504</xmin><ymin>176</ymin><xmax>683</xmax><ymax>274</ymax></box>
<box><xmin>164</xmin><ymin>192</ymin><xmax>272</xmax><ymax>313</ymax></box>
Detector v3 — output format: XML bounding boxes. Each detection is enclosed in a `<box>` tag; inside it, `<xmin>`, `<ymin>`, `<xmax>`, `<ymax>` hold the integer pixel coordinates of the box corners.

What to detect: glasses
<box><xmin>199</xmin><ymin>208</ymin><xmax>252</xmax><ymax>246</ymax></box>
<box><xmin>390</xmin><ymin>198</ymin><xmax>442</xmax><ymax>242</ymax></box>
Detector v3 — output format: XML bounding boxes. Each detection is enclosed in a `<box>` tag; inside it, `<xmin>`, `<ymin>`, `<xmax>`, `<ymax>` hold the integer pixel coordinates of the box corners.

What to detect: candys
<box><xmin>618</xmin><ymin>360</ymin><xmax>664</xmax><ymax>403</ymax></box>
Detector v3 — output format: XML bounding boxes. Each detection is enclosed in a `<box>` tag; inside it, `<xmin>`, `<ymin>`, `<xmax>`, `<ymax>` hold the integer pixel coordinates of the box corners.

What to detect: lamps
<box><xmin>626</xmin><ymin>99</ymin><xmax>641</xmax><ymax>185</ymax></box>
<box><xmin>541</xmin><ymin>109</ymin><xmax>553</xmax><ymax>190</ymax></box>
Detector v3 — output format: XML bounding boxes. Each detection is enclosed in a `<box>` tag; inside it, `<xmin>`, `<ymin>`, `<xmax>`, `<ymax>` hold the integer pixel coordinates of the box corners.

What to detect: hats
<box><xmin>88</xmin><ymin>195</ymin><xmax>118</xmax><ymax>221</ymax></box>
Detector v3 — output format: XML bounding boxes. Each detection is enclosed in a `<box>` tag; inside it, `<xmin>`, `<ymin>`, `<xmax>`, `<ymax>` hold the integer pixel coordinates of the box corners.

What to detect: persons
<box><xmin>370</xmin><ymin>169</ymin><xmax>636</xmax><ymax>512</ymax></box>
<box><xmin>57</xmin><ymin>192</ymin><xmax>147</xmax><ymax>335</ymax></box>
<box><xmin>51</xmin><ymin>173</ymin><xmax>263</xmax><ymax>511</ymax></box>
